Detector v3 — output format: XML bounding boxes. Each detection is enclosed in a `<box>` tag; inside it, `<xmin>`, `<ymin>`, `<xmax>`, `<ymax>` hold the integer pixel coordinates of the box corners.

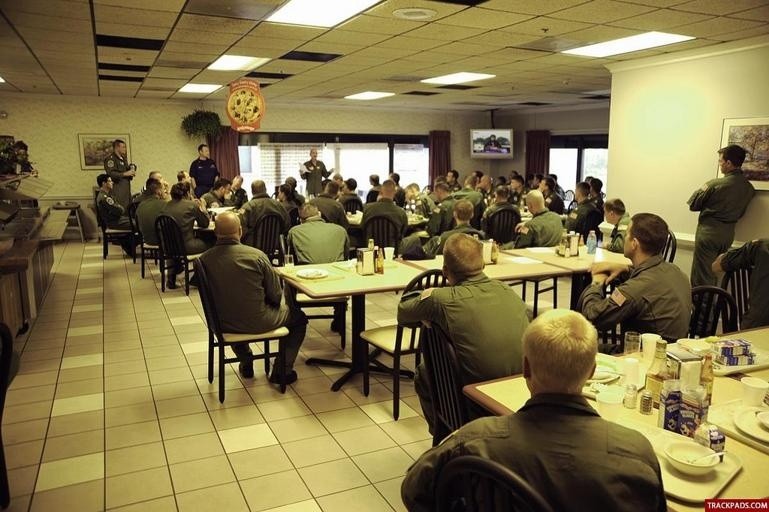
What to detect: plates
<box><xmin>578</xmin><ymin>357</ymin><xmax>627</xmax><ymax>386</ymax></box>
<box><xmin>295</xmin><ymin>269</ymin><xmax>330</xmax><ymax>278</ymax></box>
<box><xmin>733</xmin><ymin>406</ymin><xmax>769</xmax><ymax>448</ymax></box>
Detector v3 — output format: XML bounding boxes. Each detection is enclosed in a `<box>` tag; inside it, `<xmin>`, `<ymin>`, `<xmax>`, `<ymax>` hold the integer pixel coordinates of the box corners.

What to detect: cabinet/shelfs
<box><xmin>0</xmin><ymin>135</ymin><xmax>73</xmax><ymax>338</ymax></box>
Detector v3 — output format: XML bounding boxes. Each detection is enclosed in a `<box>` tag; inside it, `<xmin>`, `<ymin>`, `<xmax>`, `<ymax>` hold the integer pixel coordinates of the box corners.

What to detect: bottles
<box><xmin>554</xmin><ymin>227</ymin><xmax>597</xmax><ymax>259</ymax></box>
<box><xmin>621</xmin><ymin>330</ymin><xmax>671</xmax><ymax>417</ymax></box>
<box><xmin>368</xmin><ymin>238</ymin><xmax>385</xmax><ymax>275</ymax></box>
<box><xmin>490</xmin><ymin>239</ymin><xmax>500</xmax><ymax>264</ymax></box>
<box><xmin>701</xmin><ymin>354</ymin><xmax>714</xmax><ymax>405</ymax></box>
<box><xmin>404</xmin><ymin>194</ymin><xmax>424</xmax><ymax>218</ymax></box>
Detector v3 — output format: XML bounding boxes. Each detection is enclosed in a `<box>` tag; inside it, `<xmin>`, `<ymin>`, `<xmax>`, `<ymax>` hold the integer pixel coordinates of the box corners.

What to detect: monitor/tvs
<box><xmin>469</xmin><ymin>129</ymin><xmax>515</xmax><ymax>159</ymax></box>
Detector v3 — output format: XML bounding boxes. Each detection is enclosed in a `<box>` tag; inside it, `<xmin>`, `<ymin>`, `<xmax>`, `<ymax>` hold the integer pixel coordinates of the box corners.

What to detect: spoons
<box><xmin>678</xmin><ymin>449</ymin><xmax>728</xmax><ymax>469</ymax></box>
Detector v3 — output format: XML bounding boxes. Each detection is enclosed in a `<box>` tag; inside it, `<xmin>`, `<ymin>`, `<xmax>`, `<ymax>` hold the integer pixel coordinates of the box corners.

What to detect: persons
<box><xmin>582</xmin><ymin>213</ymin><xmax>693</xmax><ymax>356</ymax></box>
<box><xmin>686</xmin><ymin>144</ymin><xmax>755</xmax><ymax>335</ymax></box>
<box><xmin>484</xmin><ymin>134</ymin><xmax>501</xmax><ymax>149</ymax></box>
<box><xmin>600</xmin><ymin>198</ymin><xmax>632</xmax><ymax>253</ymax></box>
<box><xmin>711</xmin><ymin>237</ymin><xmax>769</xmax><ymax>330</ymax></box>
<box><xmin>400</xmin><ymin>310</ymin><xmax>668</xmax><ymax>511</ymax></box>
<box><xmin>0</xmin><ymin>140</ymin><xmax>28</xmax><ymax>176</ymax></box>
<box><xmin>395</xmin><ymin>233</ymin><xmax>531</xmax><ymax>435</ymax></box>
<box><xmin>197</xmin><ymin>212</ymin><xmax>309</xmax><ymax>384</ymax></box>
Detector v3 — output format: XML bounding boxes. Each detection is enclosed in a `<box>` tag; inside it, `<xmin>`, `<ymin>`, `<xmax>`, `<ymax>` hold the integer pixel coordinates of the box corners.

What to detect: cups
<box><xmin>595</xmin><ymin>392</ymin><xmax>624</xmax><ymax>424</ymax></box>
<box><xmin>283</xmin><ymin>254</ymin><xmax>295</xmax><ymax>273</ymax></box>
<box><xmin>740</xmin><ymin>377</ymin><xmax>769</xmax><ymax>408</ymax></box>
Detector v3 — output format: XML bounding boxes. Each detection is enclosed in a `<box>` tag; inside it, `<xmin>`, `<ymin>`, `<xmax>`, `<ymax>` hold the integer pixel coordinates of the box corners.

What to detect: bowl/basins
<box><xmin>665</xmin><ymin>439</ymin><xmax>723</xmax><ymax>477</ymax></box>
<box><xmin>757</xmin><ymin>409</ymin><xmax>769</xmax><ymax>430</ymax></box>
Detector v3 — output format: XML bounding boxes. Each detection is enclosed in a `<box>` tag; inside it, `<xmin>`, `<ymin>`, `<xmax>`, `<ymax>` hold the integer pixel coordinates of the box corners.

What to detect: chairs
<box><xmin>87</xmin><ymin>178</ymin><xmax>768</xmax><ymax>512</ymax></box>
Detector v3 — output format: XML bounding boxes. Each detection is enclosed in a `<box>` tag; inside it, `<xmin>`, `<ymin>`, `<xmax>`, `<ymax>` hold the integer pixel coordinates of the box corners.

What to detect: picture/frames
<box><xmin>76</xmin><ymin>131</ymin><xmax>134</xmax><ymax>172</ymax></box>
<box><xmin>712</xmin><ymin>114</ymin><xmax>769</xmax><ymax>192</ymax></box>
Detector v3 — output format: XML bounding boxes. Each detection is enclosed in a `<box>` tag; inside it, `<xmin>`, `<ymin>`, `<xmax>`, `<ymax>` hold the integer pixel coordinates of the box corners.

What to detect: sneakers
<box><xmin>166</xmin><ymin>274</ymin><xmax>200</xmax><ymax>290</ymax></box>
<box><xmin>237</xmin><ymin>363</ymin><xmax>258</xmax><ymax>380</ymax></box>
<box><xmin>269</xmin><ymin>367</ymin><xmax>299</xmax><ymax>385</ymax></box>
<box><xmin>121</xmin><ymin>239</ymin><xmax>133</xmax><ymax>258</ymax></box>
<box><xmin>330</xmin><ymin>320</ymin><xmax>341</xmax><ymax>335</ymax></box>
